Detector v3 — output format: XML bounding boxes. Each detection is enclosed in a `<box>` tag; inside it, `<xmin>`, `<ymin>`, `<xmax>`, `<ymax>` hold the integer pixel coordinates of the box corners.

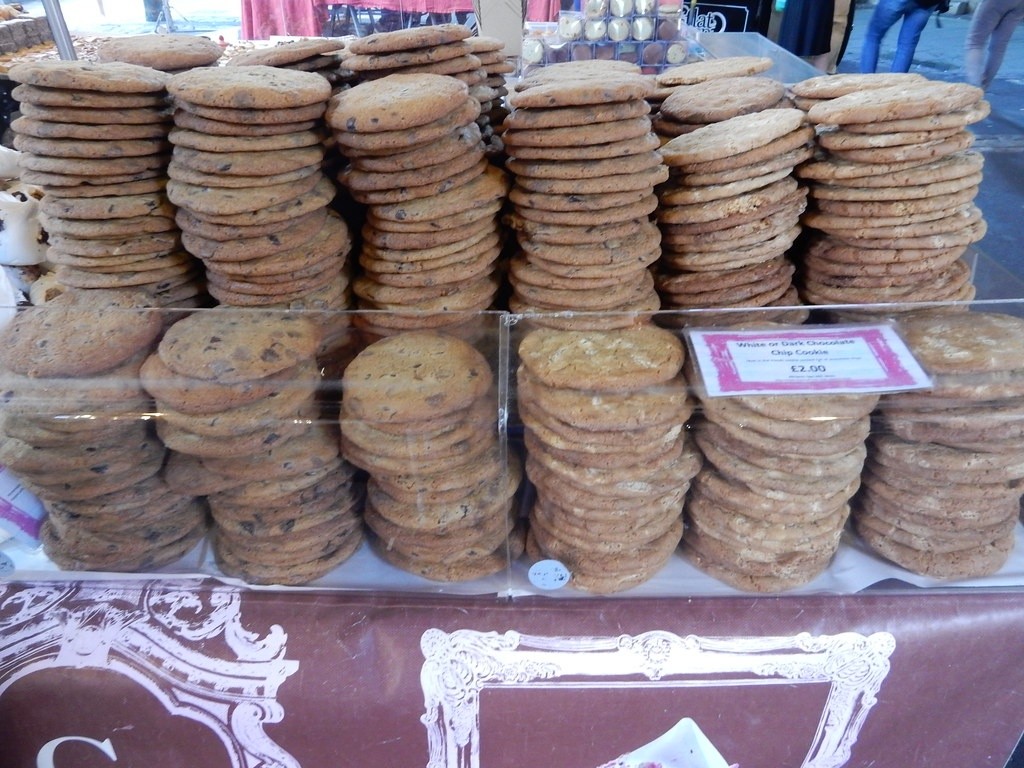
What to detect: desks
<box><xmin>0</xmin><ymin>585</ymin><xmax>1024</xmax><ymax>768</ymax></box>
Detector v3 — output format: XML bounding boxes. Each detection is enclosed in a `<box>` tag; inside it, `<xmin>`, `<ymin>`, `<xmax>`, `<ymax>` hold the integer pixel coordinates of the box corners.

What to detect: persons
<box><xmin>966</xmin><ymin>0</ymin><xmax>1024</xmax><ymax>90</ymax></box>
<box><xmin>778</xmin><ymin>0</ymin><xmax>858</xmax><ymax>75</ymax></box>
<box><xmin>683</xmin><ymin>0</ymin><xmax>772</xmax><ymax>38</ymax></box>
<box><xmin>862</xmin><ymin>0</ymin><xmax>938</xmax><ymax>73</ymax></box>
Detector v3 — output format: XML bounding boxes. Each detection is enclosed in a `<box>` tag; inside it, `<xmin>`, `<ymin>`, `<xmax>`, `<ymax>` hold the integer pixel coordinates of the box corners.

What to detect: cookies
<box><xmin>0</xmin><ymin>23</ymin><xmax>1024</xmax><ymax>594</ymax></box>
